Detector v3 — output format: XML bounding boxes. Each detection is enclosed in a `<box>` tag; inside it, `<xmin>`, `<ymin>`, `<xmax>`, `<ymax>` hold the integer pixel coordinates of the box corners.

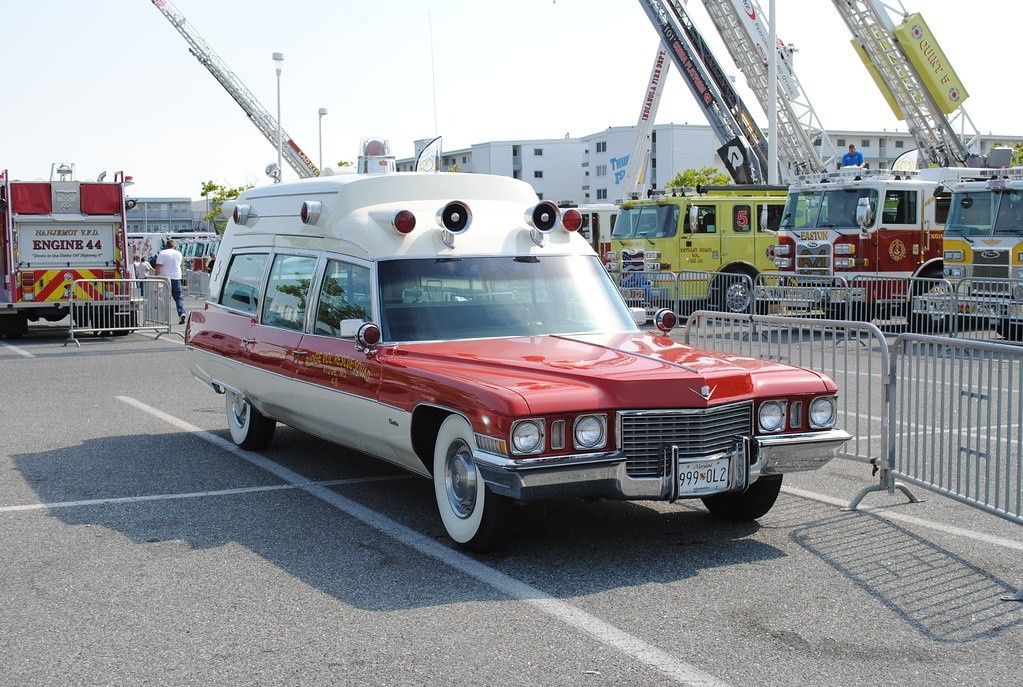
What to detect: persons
<box><xmin>133</xmin><ymin>254</ymin><xmax>157</xmax><ymax>296</ymax></box>
<box><xmin>842</xmin><ymin>144</ymin><xmax>865</xmax><ymax>168</ymax></box>
<box><xmin>206</xmin><ymin>253</ymin><xmax>216</xmax><ymax>272</ymax></box>
<box><xmin>155</xmin><ymin>240</ymin><xmax>186</xmax><ymax>324</ymax></box>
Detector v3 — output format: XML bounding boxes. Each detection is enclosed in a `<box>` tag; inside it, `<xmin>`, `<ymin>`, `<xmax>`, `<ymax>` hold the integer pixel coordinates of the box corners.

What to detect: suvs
<box><xmin>182</xmin><ymin>171</ymin><xmax>854</xmax><ymax>554</ymax></box>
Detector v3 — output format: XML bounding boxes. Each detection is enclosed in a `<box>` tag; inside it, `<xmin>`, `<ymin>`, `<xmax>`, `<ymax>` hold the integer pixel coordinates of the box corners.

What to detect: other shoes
<box><xmin>179</xmin><ymin>314</ymin><xmax>185</xmax><ymax>324</ymax></box>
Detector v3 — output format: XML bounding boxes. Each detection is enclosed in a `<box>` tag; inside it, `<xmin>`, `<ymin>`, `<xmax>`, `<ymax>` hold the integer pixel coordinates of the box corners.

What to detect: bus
<box><xmin>127</xmin><ymin>232</ymin><xmax>216</xmax><ymax>263</ymax></box>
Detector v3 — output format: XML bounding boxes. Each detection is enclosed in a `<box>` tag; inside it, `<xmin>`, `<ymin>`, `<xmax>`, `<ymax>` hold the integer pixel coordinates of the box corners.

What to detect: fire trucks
<box><xmin>930</xmin><ymin>168</ymin><xmax>1022</xmax><ymax>340</ymax></box>
<box><xmin>419</xmin><ymin>202</ymin><xmax>655</xmax><ymax>287</ymax></box>
<box><xmin>605</xmin><ymin>0</ymin><xmax>847</xmax><ymax>319</ymax></box>
<box><xmin>0</xmin><ymin>163</ymin><xmax>139</xmax><ymax>339</ymax></box>
<box><xmin>172</xmin><ymin>238</ymin><xmax>221</xmax><ymax>286</ymax></box>
<box><xmin>743</xmin><ymin>0</ymin><xmax>1016</xmax><ymax>334</ymax></box>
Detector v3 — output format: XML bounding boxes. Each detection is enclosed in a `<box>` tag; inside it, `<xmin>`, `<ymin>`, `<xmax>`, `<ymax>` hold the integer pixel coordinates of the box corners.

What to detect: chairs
<box><xmin>702</xmin><ymin>213</ymin><xmax>716</xmax><ymax>234</ymax></box>
<box><xmin>683</xmin><ymin>214</ymin><xmax>699</xmax><ymax>234</ymax></box>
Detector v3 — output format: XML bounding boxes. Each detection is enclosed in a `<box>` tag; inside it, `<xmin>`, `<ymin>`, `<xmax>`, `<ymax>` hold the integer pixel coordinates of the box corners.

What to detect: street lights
<box><xmin>270</xmin><ymin>51</ymin><xmax>286</xmax><ymax>182</ymax></box>
<box><xmin>318</xmin><ymin>106</ymin><xmax>328</xmax><ymax>176</ymax></box>
